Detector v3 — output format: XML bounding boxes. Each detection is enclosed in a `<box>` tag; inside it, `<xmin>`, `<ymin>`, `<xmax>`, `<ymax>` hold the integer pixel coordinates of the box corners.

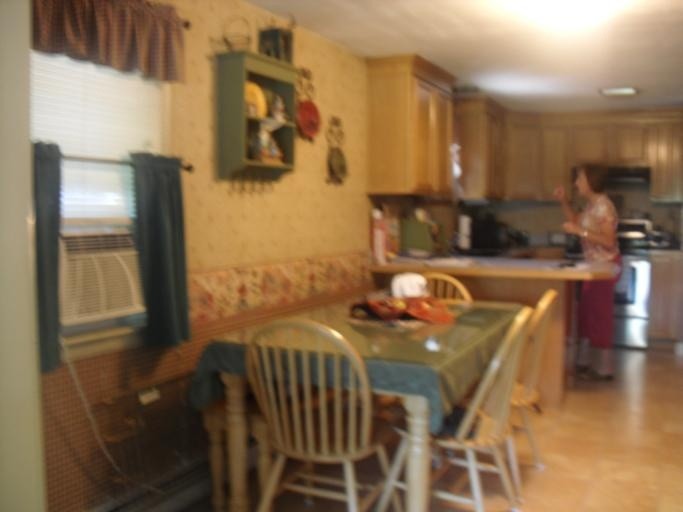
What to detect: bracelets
<box><xmin>579</xmin><ymin>227</ymin><xmax>589</xmax><ymax>238</ymax></box>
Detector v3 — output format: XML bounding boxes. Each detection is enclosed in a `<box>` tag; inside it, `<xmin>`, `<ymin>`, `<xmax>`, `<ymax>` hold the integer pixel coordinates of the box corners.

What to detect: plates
<box><xmin>297</xmin><ymin>102</ymin><xmax>320</xmax><ymax>139</ymax></box>
<box><xmin>330</xmin><ymin>147</ymin><xmax>347</xmax><ymax>182</ymax></box>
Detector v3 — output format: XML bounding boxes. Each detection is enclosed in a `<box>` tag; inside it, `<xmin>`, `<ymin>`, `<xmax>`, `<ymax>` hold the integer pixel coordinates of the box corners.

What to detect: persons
<box><xmin>551</xmin><ymin>161</ymin><xmax>624</xmax><ymax>383</ymax></box>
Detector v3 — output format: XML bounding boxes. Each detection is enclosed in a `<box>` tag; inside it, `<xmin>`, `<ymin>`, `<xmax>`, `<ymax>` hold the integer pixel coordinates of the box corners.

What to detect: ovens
<box><xmin>613</xmin><ymin>251</ymin><xmax>653</xmax><ymax>351</ymax></box>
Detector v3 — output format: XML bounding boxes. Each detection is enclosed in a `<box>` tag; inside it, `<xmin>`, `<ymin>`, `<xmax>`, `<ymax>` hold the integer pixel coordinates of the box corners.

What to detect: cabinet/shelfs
<box><xmin>367</xmin><ymin>64</ymin><xmax>453</xmax><ymax>201</ymax></box>
<box><xmin>453</xmin><ymin>100</ymin><xmax>683</xmax><ymax>203</ymax></box>
<box><xmin>214</xmin><ymin>49</ymin><xmax>300</xmax><ymax>180</ymax></box>
<box><xmin>649</xmin><ymin>254</ymin><xmax>682</xmax><ymax>341</ymax></box>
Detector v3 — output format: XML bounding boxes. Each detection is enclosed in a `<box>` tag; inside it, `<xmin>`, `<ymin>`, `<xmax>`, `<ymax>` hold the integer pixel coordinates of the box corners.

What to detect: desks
<box><xmin>201</xmin><ymin>292</ymin><xmax>523</xmax><ymax>512</ymax></box>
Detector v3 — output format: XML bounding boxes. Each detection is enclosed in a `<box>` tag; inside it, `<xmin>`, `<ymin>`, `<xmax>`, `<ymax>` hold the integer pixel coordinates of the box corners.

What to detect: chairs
<box><xmin>244</xmin><ymin>317</ymin><xmax>409</xmax><ymax>512</ymax></box>
<box><xmin>374</xmin><ymin>272</ymin><xmax>559</xmax><ymax>512</ymax></box>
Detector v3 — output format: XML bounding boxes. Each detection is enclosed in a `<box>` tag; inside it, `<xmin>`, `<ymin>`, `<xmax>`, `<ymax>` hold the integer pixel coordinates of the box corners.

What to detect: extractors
<box><xmin>572</xmin><ymin>165</ymin><xmax>651</xmax><ymax>189</ymax></box>
